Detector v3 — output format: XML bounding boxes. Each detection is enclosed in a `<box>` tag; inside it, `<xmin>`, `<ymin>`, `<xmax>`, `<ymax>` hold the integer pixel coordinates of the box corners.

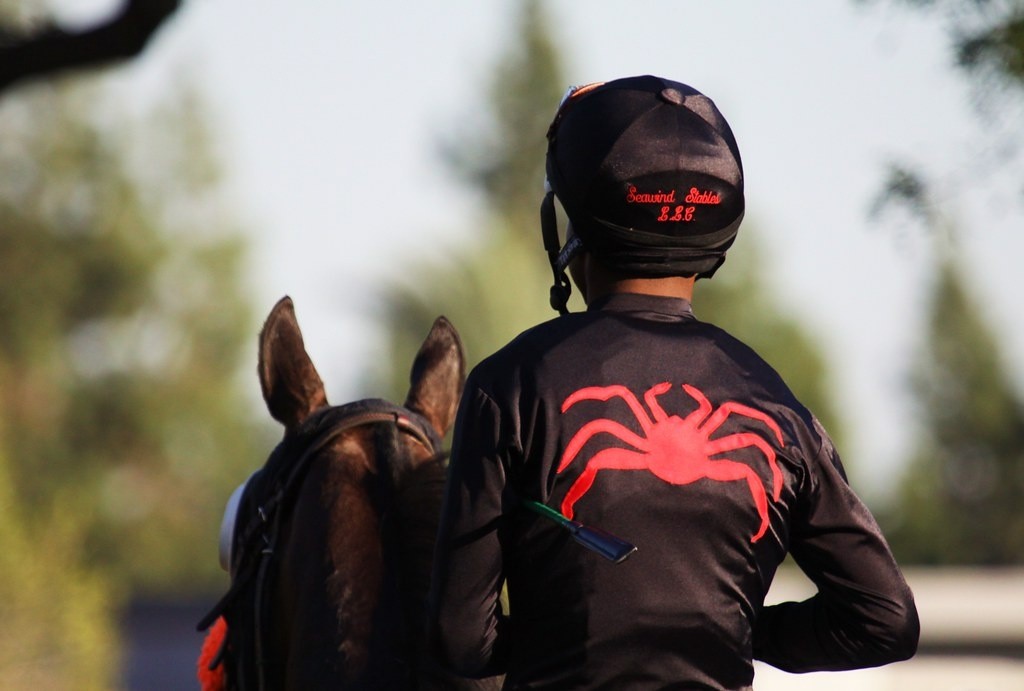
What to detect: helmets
<box><xmin>545</xmin><ymin>75</ymin><xmax>745</xmax><ymax>277</ymax></box>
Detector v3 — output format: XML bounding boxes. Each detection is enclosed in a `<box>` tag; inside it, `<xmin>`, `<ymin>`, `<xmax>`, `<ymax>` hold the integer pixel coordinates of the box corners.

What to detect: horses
<box><xmin>214</xmin><ymin>294</ymin><xmax>502</xmax><ymax>691</ymax></box>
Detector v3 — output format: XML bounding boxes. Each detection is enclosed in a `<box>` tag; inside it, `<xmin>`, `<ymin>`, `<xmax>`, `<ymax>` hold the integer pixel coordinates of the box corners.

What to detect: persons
<box><xmin>438</xmin><ymin>76</ymin><xmax>921</xmax><ymax>691</ymax></box>
